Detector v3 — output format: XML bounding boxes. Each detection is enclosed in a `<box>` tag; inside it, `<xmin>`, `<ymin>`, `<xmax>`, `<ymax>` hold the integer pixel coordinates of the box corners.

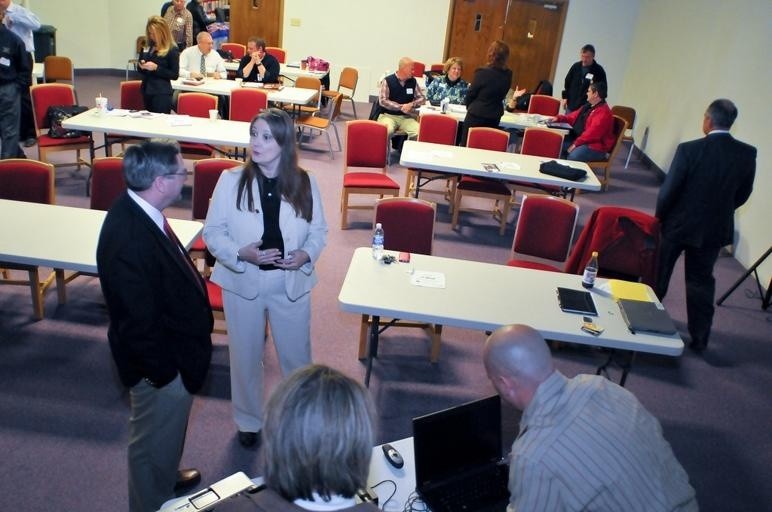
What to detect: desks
<box><xmin>156</xmin><ymin>432</ymin><xmax>429</xmax><ymax>512</ymax></box>
<box><xmin>1</xmin><ymin>199</ymin><xmax>230</xmax><ymax>336</ymax></box>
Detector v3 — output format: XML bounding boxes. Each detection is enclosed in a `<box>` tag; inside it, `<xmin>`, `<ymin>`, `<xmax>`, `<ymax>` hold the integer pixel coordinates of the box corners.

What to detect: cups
<box><xmin>440</xmin><ymin>100</ymin><xmax>447</xmax><ymax>112</ymax></box>
<box><xmin>235</xmin><ymin>78</ymin><xmax>243</xmax><ymax>88</ymax></box>
<box><xmin>95</xmin><ymin>96</ymin><xmax>107</xmax><ymax>115</ymax></box>
<box><xmin>209</xmin><ymin>109</ymin><xmax>218</xmax><ymax>122</ymax></box>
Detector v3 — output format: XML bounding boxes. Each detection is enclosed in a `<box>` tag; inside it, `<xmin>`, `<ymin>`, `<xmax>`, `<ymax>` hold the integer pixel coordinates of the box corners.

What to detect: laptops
<box><xmin>414</xmin><ymin>394</ymin><xmax>511</xmax><ymax>511</ymax></box>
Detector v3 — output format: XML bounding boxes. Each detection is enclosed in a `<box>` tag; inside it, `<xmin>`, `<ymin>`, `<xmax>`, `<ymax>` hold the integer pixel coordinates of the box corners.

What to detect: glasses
<box><xmin>151</xmin><ymin>170</ymin><xmax>193</xmax><ymax>184</ymax></box>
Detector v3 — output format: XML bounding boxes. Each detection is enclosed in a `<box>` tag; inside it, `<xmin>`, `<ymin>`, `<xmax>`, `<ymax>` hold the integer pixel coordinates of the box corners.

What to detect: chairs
<box><xmin>1</xmin><ymin>159</ymin><xmax>66</xmax><ymax>319</ymax></box>
<box><xmin>194</xmin><ymin>160</ymin><xmax>246</xmax><ymax>220</ymax></box>
<box><xmin>30</xmin><ymin>83</ymin><xmax>94</xmax><ymax>167</ymax></box>
<box><xmin>372</xmin><ymin>62</ymin><xmax>635</xmax><ymax>237</ymax></box>
<box><xmin>44</xmin><ymin>56</ymin><xmax>73</xmax><ymax>81</ymax></box>
<box><xmin>61</xmin><ymin>43</ymin><xmax>358</xmax><ymax>159</ymax></box>
<box><xmin>338</xmin><ymin>194</ymin><xmax>684</xmax><ymax>388</ymax></box>
<box><xmin>91</xmin><ymin>158</ymin><xmax>124</xmax><ymax>209</ymax></box>
<box><xmin>339</xmin><ymin>120</ymin><xmax>400</xmax><ymax>229</ymax></box>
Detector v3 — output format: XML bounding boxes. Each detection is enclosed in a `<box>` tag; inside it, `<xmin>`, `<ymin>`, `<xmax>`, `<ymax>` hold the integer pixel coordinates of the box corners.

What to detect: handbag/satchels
<box><xmin>540</xmin><ymin>161</ymin><xmax>587</xmax><ymax>181</ymax></box>
<box><xmin>308</xmin><ymin>56</ymin><xmax>329</xmax><ymax>71</ymax></box>
<box><xmin>48</xmin><ymin>106</ymin><xmax>90</xmax><ymax>138</ymax></box>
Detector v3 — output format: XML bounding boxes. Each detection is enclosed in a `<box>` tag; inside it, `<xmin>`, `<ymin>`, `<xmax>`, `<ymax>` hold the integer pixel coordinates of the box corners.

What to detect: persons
<box><xmin>202</xmin><ymin>109</ymin><xmax>327</xmax><ymax>448</ymax></box>
<box><xmin>0</xmin><ymin>0</ymin><xmax>43</xmax><ymax>161</ymax></box>
<box><xmin>203</xmin><ymin>366</ymin><xmax>385</xmax><ymax>512</ymax></box>
<box><xmin>95</xmin><ymin>140</ymin><xmax>214</xmax><ymax>511</ymax></box>
<box><xmin>379</xmin><ymin>37</ymin><xmax>615</xmax><ymax>194</ymax></box>
<box><xmin>653</xmin><ymin>101</ymin><xmax>757</xmax><ymax>354</ymax></box>
<box><xmin>137</xmin><ymin>0</ymin><xmax>280</xmax><ymax>113</ymax></box>
<box><xmin>480</xmin><ymin>324</ymin><xmax>700</xmax><ymax>511</ymax></box>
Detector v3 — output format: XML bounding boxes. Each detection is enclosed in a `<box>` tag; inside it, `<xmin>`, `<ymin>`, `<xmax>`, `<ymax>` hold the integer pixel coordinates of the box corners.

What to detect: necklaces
<box><xmin>262</xmin><ymin>178</ymin><xmax>279</xmax><ymax>197</ymax></box>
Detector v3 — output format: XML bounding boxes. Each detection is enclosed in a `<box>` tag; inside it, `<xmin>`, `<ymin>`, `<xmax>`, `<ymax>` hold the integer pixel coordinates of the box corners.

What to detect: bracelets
<box><xmin>236</xmin><ymin>249</ymin><xmax>240</xmax><ymax>259</ymax></box>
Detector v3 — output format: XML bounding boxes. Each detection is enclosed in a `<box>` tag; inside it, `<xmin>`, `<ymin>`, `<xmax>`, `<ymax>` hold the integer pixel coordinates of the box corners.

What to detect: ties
<box><xmin>200</xmin><ymin>55</ymin><xmax>206</xmax><ymax>77</ymax></box>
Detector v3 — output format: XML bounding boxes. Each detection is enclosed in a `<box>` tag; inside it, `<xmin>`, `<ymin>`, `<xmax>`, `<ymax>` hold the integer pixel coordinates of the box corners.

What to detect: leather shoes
<box><xmin>26</xmin><ymin>137</ymin><xmax>36</xmax><ymax>146</ymax></box>
<box><xmin>687</xmin><ymin>341</ymin><xmax>708</xmax><ymax>353</ymax></box>
<box><xmin>239</xmin><ymin>432</ymin><xmax>256</xmax><ymax>447</ymax></box>
<box><xmin>174</xmin><ymin>469</ymin><xmax>201</xmax><ymax>491</ymax></box>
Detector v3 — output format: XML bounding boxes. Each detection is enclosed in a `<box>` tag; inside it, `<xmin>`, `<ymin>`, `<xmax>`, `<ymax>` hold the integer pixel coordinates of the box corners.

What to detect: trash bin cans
<box><xmin>32</xmin><ymin>25</ymin><xmax>57</xmax><ymax>84</ymax></box>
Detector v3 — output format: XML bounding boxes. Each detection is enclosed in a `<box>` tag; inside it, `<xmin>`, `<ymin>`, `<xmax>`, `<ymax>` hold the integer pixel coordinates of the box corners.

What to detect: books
<box><xmin>557</xmin><ymin>287</ymin><xmax>598</xmax><ymax>315</ymax></box>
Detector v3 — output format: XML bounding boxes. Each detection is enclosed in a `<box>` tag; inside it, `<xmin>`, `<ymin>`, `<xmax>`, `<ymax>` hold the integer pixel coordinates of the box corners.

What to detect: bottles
<box><xmin>372</xmin><ymin>223</ymin><xmax>386</xmax><ymax>261</ymax></box>
<box><xmin>581</xmin><ymin>250</ymin><xmax>600</xmax><ymax>289</ymax></box>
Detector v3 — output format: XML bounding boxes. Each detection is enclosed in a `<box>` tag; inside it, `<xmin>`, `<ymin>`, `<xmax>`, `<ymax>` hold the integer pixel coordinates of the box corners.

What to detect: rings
<box><xmin>259</xmin><ymin>250</ymin><xmax>266</xmax><ymax>256</ymax></box>
